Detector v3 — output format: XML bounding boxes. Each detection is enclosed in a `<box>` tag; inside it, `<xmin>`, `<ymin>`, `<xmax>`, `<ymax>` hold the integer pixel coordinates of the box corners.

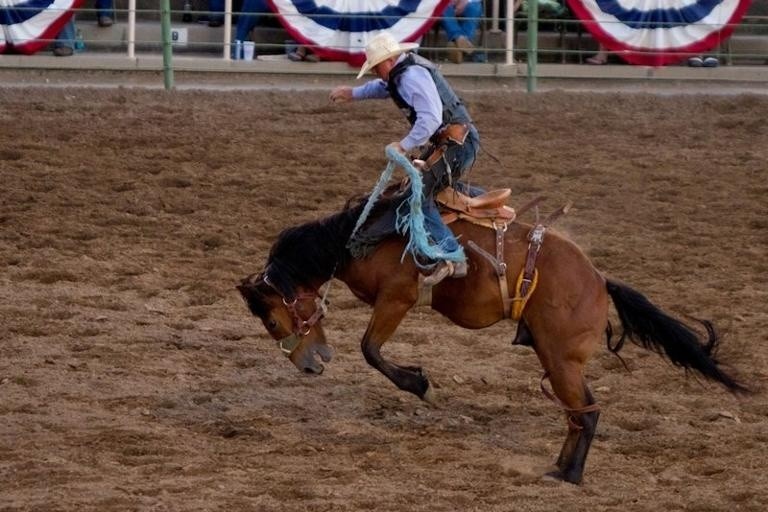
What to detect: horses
<box><xmin>235</xmin><ymin>182</ymin><xmax>753</xmax><ymax>492</ymax></box>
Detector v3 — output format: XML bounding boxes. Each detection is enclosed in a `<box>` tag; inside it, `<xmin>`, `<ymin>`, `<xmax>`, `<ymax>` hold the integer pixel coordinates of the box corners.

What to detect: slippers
<box><xmin>288</xmin><ymin>51</ymin><xmax>321</xmax><ymax>62</ymax></box>
<box><xmin>586</xmin><ymin>56</ymin><xmax>609</xmax><ymax>65</ymax></box>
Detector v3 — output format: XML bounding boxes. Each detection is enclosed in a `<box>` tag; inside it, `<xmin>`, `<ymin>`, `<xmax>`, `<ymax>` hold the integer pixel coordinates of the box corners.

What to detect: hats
<box><xmin>356</xmin><ymin>32</ymin><xmax>419</xmax><ymax>79</ymax></box>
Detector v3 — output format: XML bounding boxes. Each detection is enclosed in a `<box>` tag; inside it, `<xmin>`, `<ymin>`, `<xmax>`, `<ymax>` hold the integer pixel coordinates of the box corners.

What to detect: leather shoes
<box><xmin>53</xmin><ymin>47</ymin><xmax>73</xmax><ymax>55</ymax></box>
<box><xmin>210</xmin><ymin>18</ymin><xmax>224</xmax><ymax>27</ymax></box>
<box><xmin>423</xmin><ymin>254</ymin><xmax>467</xmax><ymax>286</ymax></box>
<box><xmin>98</xmin><ymin>15</ymin><xmax>114</xmax><ymax>27</ymax></box>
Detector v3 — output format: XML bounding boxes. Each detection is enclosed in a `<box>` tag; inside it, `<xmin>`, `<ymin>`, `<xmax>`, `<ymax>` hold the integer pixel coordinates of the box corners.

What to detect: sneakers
<box><xmin>446</xmin><ymin>37</ymin><xmax>476</xmax><ymax>63</ymax></box>
<box><xmin>689</xmin><ymin>56</ymin><xmax>719</xmax><ymax>67</ymax></box>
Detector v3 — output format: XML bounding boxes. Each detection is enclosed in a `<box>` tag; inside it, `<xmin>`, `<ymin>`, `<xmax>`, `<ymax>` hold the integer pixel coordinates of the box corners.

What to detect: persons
<box><xmin>328</xmin><ymin>30</ymin><xmax>482</xmax><ymax>288</ymax></box>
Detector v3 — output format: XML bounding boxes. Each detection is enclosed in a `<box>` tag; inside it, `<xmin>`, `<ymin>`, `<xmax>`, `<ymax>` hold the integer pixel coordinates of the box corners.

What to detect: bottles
<box><xmin>75</xmin><ymin>26</ymin><xmax>85</xmax><ymax>50</ymax></box>
